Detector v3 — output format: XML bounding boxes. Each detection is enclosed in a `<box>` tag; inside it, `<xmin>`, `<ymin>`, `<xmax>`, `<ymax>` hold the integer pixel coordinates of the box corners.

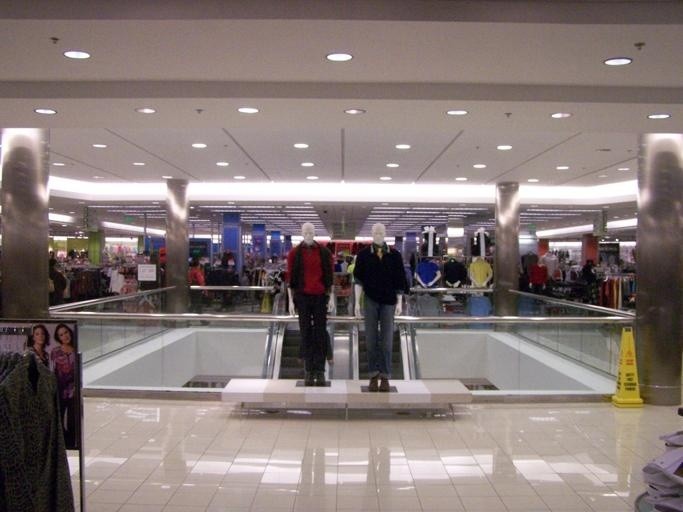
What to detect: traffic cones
<box><xmin>606</xmin><ymin>325</ymin><xmax>644</xmax><ymax>408</ymax></box>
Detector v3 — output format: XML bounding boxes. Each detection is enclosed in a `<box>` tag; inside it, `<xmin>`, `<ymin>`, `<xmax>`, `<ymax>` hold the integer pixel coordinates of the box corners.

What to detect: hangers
<box><xmin>0</xmin><ymin>327</ymin><xmax>35</xmax><ymax>367</ymax></box>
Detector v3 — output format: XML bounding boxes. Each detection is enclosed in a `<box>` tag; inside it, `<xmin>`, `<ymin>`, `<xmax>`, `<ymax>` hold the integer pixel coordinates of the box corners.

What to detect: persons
<box><xmin>443</xmin><ymin>259</ymin><xmax>465</xmax><ymax>289</ymax></box>
<box><xmin>285</xmin><ymin>223</ymin><xmax>335</xmax><ymax>386</ymax></box>
<box><xmin>467</xmin><ymin>253</ymin><xmax>493</xmax><ymax>297</ymax></box>
<box><xmin>353</xmin><ymin>223</ymin><xmax>407</xmax><ymax>392</ymax></box>
<box><xmin>51</xmin><ymin>324</ymin><xmax>75</xmax><ymax>449</ymax></box>
<box><xmin>24</xmin><ymin>326</ymin><xmax>49</xmax><ymax>368</ymax></box>
<box><xmin>188</xmin><ymin>257</ymin><xmax>209</xmax><ymax>325</ymax></box>
<box><xmin>414</xmin><ymin>259</ymin><xmax>441</xmax><ymax>287</ymax></box>
<box><xmin>528</xmin><ymin>263</ymin><xmax>548</xmax><ymax>293</ymax></box>
<box><xmin>49</xmin><ymin>258</ymin><xmax>67</xmax><ymax>306</ymax></box>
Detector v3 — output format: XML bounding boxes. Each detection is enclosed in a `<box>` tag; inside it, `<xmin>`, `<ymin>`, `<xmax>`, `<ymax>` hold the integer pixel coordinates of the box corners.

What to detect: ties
<box><xmin>377</xmin><ymin>248</ymin><xmax>383</xmax><ymax>259</ymax></box>
